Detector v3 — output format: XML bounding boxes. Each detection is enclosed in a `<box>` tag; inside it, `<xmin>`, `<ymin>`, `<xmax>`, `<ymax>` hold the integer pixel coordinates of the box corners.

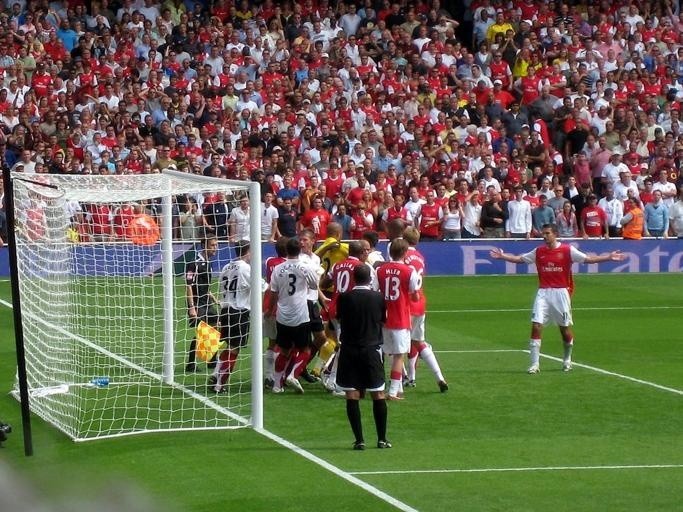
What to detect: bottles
<box><xmin>89</xmin><ymin>378</ymin><xmax>109</xmax><ymax>387</ymax></box>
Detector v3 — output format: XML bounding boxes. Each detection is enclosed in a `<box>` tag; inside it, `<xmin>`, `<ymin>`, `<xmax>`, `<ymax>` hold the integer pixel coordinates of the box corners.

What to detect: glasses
<box><xmin>449</xmin><ymin>200</ymin><xmax>455</xmax><ymax>202</ymax></box>
<box><xmin>25</xmin><ymin>17</ymin><xmax>34</xmax><ymax>20</ymax></box>
<box><xmin>192</xmin><ymin>91</ymin><xmax>358</xmax><ymax>175</ymax></box>
<box><xmin>555</xmin><ymin>189</ymin><xmax>562</xmax><ymax>192</ymax></box>
<box><xmin>115</xmin><ymin>164</ymin><xmax>124</xmax><ymax>167</ymax></box>
<box><xmin>388</xmin><ymin>168</ymin><xmax>394</xmax><ymax>171</ymax></box>
<box><xmin>149</xmin><ymin>53</ymin><xmax>231</xmax><ymax>100</ymax></box>
<box><xmin>493</xmin><ymin>54</ymin><xmax>501</xmax><ymax>57</ymax></box>
<box><xmin>500</xmin><ymin>161</ymin><xmax>506</xmax><ymax>164</ymax></box>
<box><xmin>512</xmin><ymin>104</ymin><xmax>520</xmax><ymax>107</ymax></box>
<box><xmin>163</xmin><ymin>149</ymin><xmax>171</xmax><ymax>152</ymax></box>
<box><xmin>432</xmin><ymin>70</ymin><xmax>438</xmax><ymax>73</ymax></box>
<box><xmin>263</xmin><ymin>209</ymin><xmax>268</xmax><ymax>216</ymax></box>
<box><xmin>521</xmin><ymin>160</ymin><xmax>527</xmax><ymax>163</ymax></box>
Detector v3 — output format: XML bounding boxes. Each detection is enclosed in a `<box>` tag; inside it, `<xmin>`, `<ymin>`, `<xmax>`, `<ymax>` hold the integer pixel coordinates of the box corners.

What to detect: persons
<box><xmin>335</xmin><ymin>266</ymin><xmax>392</xmax><ymax>448</ymax></box>
<box><xmin>2</xmin><ymin>0</ymin><xmax>682</xmax><ymax>243</ymax></box>
<box><xmin>488</xmin><ymin>223</ymin><xmax>630</xmax><ymax>373</ymax></box>
<box><xmin>185</xmin><ymin>220</ymin><xmax>450</xmax><ymax>398</ymax></box>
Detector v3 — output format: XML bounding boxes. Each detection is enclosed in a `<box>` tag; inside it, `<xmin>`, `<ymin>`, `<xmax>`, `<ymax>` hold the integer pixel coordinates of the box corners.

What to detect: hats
<box><xmin>492</xmin><ymin>78</ymin><xmax>502</xmax><ymax>86</ymax></box>
<box><xmin>255</xmin><ymin>168</ymin><xmax>264</xmax><ymax>174</ymax></box>
<box><xmin>523</xmin><ymin>19</ymin><xmax>533</xmax><ymax>25</ymax></box>
<box><xmin>499</xmin><ymin>156</ymin><xmax>507</xmax><ymax>162</ymax></box>
<box><xmin>351</xmin><ymin>77</ymin><xmax>363</xmax><ymax>82</ymax></box>
<box><xmin>166</xmin><ymin>163</ymin><xmax>177</xmax><ymax>170</ymax></box>
<box><xmin>36</xmin><ymin>62</ymin><xmax>46</xmax><ymax>70</ymax></box>
<box><xmin>272</xmin><ymin>145</ymin><xmax>282</xmax><ymax>152</ymax></box>
<box><xmin>302</xmin><ymin>98</ymin><xmax>310</xmax><ymax>104</ymax></box>
<box><xmin>354</xmin><ymin>163</ymin><xmax>365</xmax><ymax>170</ymax></box>
<box><xmin>588</xmin><ymin>193</ymin><xmax>596</xmax><ymax>199</ymax></box>
<box><xmin>320</xmin><ymin>52</ymin><xmax>329</xmax><ymax>58</ymax></box>
<box><xmin>628</xmin><ymin>152</ymin><xmax>639</xmax><ymax>160</ymax></box>
<box><xmin>521</xmin><ymin>123</ymin><xmax>532</xmax><ymax>130</ymax></box>
<box><xmin>639</xmin><ymin>163</ymin><xmax>649</xmax><ymax>171</ymax></box>
<box><xmin>648</xmin><ymin>37</ymin><xmax>676</xmax><ymax>44</ymax></box>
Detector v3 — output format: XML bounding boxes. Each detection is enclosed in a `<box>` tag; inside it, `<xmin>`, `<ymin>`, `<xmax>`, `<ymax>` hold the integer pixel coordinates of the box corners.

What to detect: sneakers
<box><xmin>376</xmin><ymin>439</ymin><xmax>392</xmax><ymax>449</ymax></box>
<box><xmin>560</xmin><ymin>362</ymin><xmax>573</xmax><ymax>373</ymax></box>
<box><xmin>263</xmin><ymin>369</ymin><xmax>320</xmax><ymax>396</ymax></box>
<box><xmin>184</xmin><ymin>362</ymin><xmax>203</xmax><ymax>374</ymax></box>
<box><xmin>438</xmin><ymin>378</ymin><xmax>449</xmax><ymax>392</ymax></box>
<box><xmin>352</xmin><ymin>439</ymin><xmax>366</xmax><ymax>451</ymax></box>
<box><xmin>207</xmin><ymin>376</ymin><xmax>228</xmax><ymax>395</ymax></box>
<box><xmin>401</xmin><ymin>374</ymin><xmax>416</xmax><ymax>389</ymax></box>
<box><xmin>206</xmin><ymin>359</ymin><xmax>218</xmax><ymax>369</ymax></box>
<box><xmin>526</xmin><ymin>366</ymin><xmax>541</xmax><ymax>375</ymax></box>
<box><xmin>383</xmin><ymin>389</ymin><xmax>405</xmax><ymax>402</ymax></box>
<box><xmin>321</xmin><ymin>373</ymin><xmax>347</xmax><ymax>397</ymax></box>
